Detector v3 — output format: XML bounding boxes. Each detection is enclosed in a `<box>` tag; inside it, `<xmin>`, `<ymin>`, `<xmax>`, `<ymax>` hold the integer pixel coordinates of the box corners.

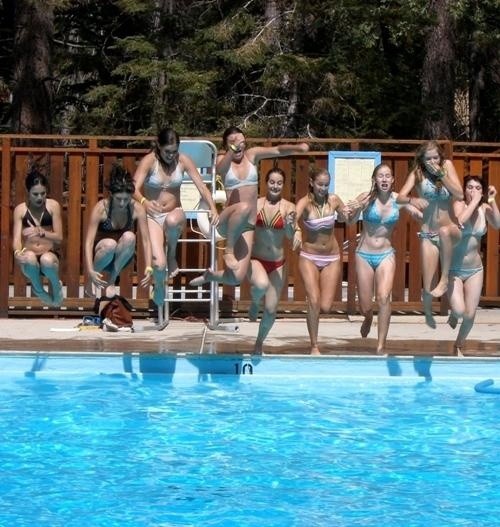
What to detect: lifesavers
<box><xmin>197</xmin><ymin>188</ymin><xmax>227</xmax><ymax>244</ymax></box>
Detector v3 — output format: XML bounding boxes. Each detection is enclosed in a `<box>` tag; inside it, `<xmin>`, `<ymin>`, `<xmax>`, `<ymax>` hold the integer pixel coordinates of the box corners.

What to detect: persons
<box><xmin>188</xmin><ymin>126</ymin><xmax>309</xmax><ymax>287</ymax></box>
<box><xmin>128</xmin><ymin>127</ymin><xmax>219</xmax><ymax>280</ymax></box>
<box><xmin>290</xmin><ymin>166</ymin><xmax>344</xmax><ymax>354</ymax></box>
<box><xmin>394</xmin><ymin>138</ymin><xmax>464</xmax><ymax>329</ymax></box>
<box><xmin>139</xmin><ymin>196</ymin><xmax>147</xmax><ymax>205</ymax></box>
<box><xmin>244</xmin><ymin>166</ymin><xmax>298</xmax><ymax>356</ymax></box>
<box><xmin>84</xmin><ymin>163</ymin><xmax>154</xmax><ymax>299</ymax></box>
<box><xmin>345</xmin><ymin>164</ymin><xmax>423</xmax><ymax>356</ymax></box>
<box><xmin>12</xmin><ymin>163</ymin><xmax>64</xmax><ymax>308</ymax></box>
<box><xmin>231</xmin><ymin>143</ymin><xmax>239</xmax><ymax>153</ymax></box>
<box><xmin>445</xmin><ymin>174</ymin><xmax>500</xmax><ymax>357</ymax></box>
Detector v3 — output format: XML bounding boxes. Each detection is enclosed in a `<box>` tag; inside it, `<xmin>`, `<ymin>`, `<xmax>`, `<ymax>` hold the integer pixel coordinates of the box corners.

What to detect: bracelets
<box><xmin>143</xmin><ymin>266</ymin><xmax>153</xmax><ymax>275</ymax></box>
<box><xmin>487</xmin><ymin>197</ymin><xmax>495</xmax><ymax>203</ymax></box>
<box><xmin>14</xmin><ymin>249</ymin><xmax>20</xmax><ymax>255</ymax></box>
<box><xmin>292</xmin><ymin>226</ymin><xmax>303</xmax><ymax>231</ymax></box>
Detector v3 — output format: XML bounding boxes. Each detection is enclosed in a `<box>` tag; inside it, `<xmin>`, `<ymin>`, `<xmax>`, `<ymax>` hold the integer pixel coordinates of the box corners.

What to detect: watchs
<box><xmin>40</xmin><ymin>228</ymin><xmax>45</xmax><ymax>238</ymax></box>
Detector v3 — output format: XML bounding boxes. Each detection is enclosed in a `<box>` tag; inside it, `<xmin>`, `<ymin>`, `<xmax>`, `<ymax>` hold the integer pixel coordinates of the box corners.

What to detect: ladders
<box><xmin>143</xmin><ymin>139</ymin><xmax>237</xmax><ymax>332</ymax></box>
